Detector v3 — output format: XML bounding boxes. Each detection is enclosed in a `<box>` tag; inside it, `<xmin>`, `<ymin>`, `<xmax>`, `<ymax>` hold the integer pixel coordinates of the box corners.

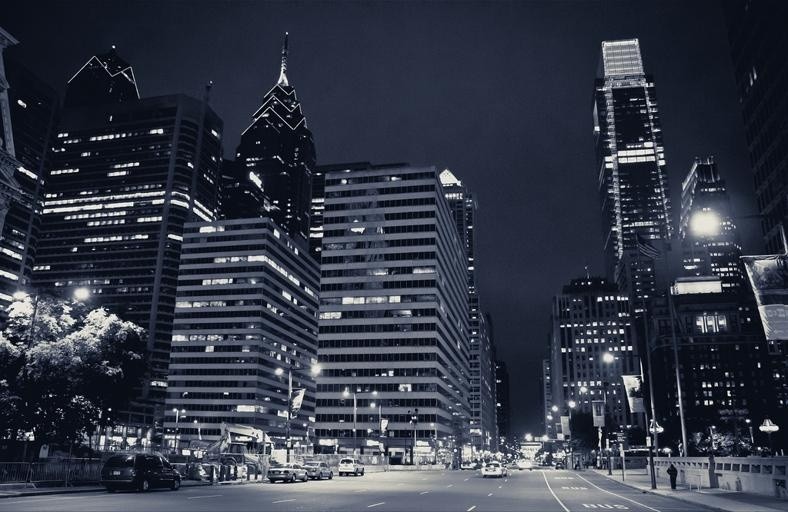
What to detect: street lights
<box><xmin>412</xmin><ymin>415</ymin><xmax>417</xmax><ymax>464</ymax></box>
<box><xmin>601</xmin><ymin>354</ymin><xmax>656</xmax><ymax>490</ymax></box>
<box><xmin>170</xmin><ymin>408</ymin><xmax>186</xmax><ymax>449</ymax></box>
<box><xmin>287</xmin><ymin>367</ymin><xmax>317</xmax><ymax>439</ymax></box>
<box><xmin>343</xmin><ymin>392</ymin><xmax>357</xmax><ymax>446</ymax></box>
<box><xmin>580</xmin><ymin>386</ymin><xmax>614</xmax><ymax>479</ymax></box>
<box><xmin>5</xmin><ymin>287</ymin><xmax>87</xmax><ymax>457</ymax></box>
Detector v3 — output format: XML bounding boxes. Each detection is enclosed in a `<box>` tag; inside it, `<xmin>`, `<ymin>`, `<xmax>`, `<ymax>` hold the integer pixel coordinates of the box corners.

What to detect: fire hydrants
<box><xmin>666</xmin><ymin>462</ymin><xmax>681</xmax><ymax>490</ymax></box>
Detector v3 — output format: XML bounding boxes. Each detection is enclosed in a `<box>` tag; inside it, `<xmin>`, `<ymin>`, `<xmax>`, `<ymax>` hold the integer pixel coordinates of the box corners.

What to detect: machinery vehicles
<box><xmin>205</xmin><ymin>423</ymin><xmax>274</xmax><ymax>479</ymax></box>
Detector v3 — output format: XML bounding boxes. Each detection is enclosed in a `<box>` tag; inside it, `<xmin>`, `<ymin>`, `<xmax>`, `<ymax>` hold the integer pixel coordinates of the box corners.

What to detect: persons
<box><xmin>667</xmin><ymin>464</ymin><xmax>677</xmax><ymax>489</ymax></box>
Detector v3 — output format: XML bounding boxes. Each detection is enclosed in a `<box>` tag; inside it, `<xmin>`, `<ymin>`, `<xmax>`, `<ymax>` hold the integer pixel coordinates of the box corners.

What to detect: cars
<box><xmin>102</xmin><ymin>453</ymin><xmax>180</xmax><ymax>491</ymax></box>
<box><xmin>460</xmin><ymin>452</ymin><xmax>565</xmax><ymax>479</ymax></box>
<box><xmin>269</xmin><ymin>458</ymin><xmax>366</xmax><ymax>483</ymax></box>
